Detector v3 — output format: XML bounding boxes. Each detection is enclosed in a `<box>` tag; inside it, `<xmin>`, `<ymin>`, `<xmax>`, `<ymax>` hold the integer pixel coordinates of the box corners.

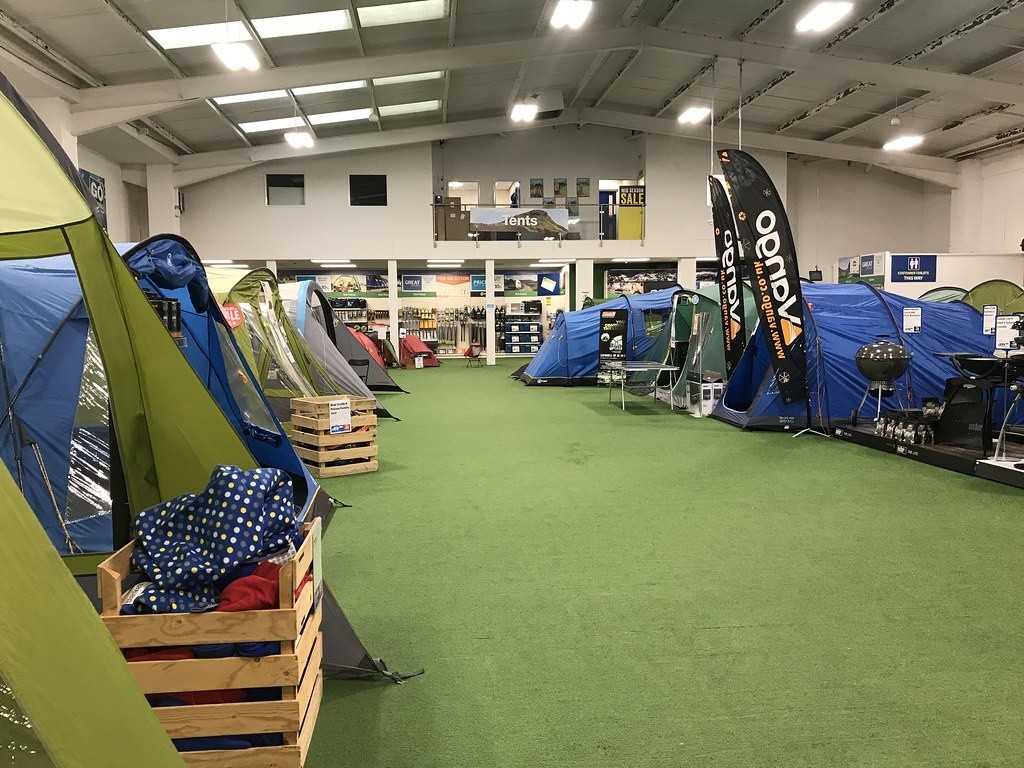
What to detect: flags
<box><xmin>717</xmin><ymin>149</ymin><xmax>809</xmax><ymax>406</ymax></box>
<box><xmin>708</xmin><ymin>177</ymin><xmax>747</xmax><ymax>380</ymax></box>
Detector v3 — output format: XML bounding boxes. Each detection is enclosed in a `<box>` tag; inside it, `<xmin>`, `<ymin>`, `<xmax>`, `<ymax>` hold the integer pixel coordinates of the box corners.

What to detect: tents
<box><xmin>0</xmin><ymin>234</ymin><xmax>351</xmax><ymax>612</ymax></box>
<box><xmin>0</xmin><ymin>73</ymin><xmax>393</xmax><ymax>768</ymax></box>
<box><xmin>627</xmin><ymin>279</ymin><xmax>759</xmax><ymax>409</ymax></box>
<box><xmin>511</xmin><ymin>284</ymin><xmax>695</xmax><ymax>386</ymax></box>
<box><xmin>347</xmin><ymin>327</ymin><xmax>441</xmax><ymax>372</ymax></box>
<box><xmin>707</xmin><ymin>279</ymin><xmax>1024</xmax><ymax>431</ymax></box>
<box><xmin>75</xmin><ymin>264</ymin><xmax>346</xmax><ymax>439</ymax></box>
<box><xmin>238</xmin><ymin>280</ymin><xmax>410</xmax><ymax>422</ymax></box>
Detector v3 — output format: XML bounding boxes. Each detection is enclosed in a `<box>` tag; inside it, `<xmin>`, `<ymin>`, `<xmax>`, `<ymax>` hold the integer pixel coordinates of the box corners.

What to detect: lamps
<box><xmin>368</xmin><ymin>89</ymin><xmax>378</xmax><ymax>122</ymax></box>
<box><xmin>890</xmin><ymin>90</ymin><xmax>900</xmax><ymax>126</ymax></box>
<box><xmin>809</xmin><ymin>268</ymin><xmax>822</xmax><ymax>281</ymax></box>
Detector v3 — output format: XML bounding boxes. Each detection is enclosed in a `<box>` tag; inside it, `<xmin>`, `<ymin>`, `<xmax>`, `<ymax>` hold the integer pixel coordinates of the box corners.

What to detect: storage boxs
<box><xmin>686</xmin><ymin>380</ymin><xmax>727</xmax><ymax>416</ymax></box>
<box><xmin>98</xmin><ymin>517</ymin><xmax>323</xmax><ymax>768</ymax></box>
<box><xmin>290</xmin><ymin>395</ymin><xmax>378</xmax><ymax>479</ymax></box>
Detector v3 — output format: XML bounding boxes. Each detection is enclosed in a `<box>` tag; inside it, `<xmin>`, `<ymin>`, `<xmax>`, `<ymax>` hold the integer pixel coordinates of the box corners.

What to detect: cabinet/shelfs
<box><xmin>445</xmin><ymin>211</ymin><xmax>470</xmax><ymax>240</ymax></box>
<box><xmin>333</xmin><ymin>308</ymin><xmax>368</xmax><ymax>323</ymax></box>
<box><xmin>505</xmin><ymin>313</ymin><xmax>540</xmax><ymax>354</ymax></box>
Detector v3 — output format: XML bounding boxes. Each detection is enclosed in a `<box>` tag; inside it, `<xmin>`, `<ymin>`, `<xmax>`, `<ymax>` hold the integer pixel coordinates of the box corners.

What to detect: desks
<box><xmin>605</xmin><ymin>361</ymin><xmax>680</xmax><ymax>411</ymax></box>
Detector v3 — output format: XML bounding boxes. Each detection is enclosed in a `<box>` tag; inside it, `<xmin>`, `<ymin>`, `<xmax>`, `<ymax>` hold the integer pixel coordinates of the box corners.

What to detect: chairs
<box><xmin>348</xmin><ymin>359</ymin><xmax>370</xmax><ymax>385</ymax></box>
<box><xmin>464</xmin><ymin>345</ymin><xmax>483</xmax><ymax>367</ymax></box>
